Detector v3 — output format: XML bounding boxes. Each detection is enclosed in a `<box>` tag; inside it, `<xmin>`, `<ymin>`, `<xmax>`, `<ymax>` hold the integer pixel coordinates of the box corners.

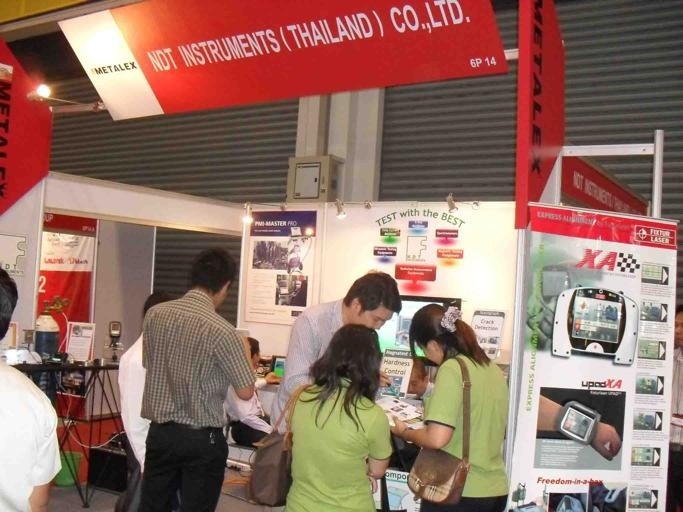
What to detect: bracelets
<box><xmin>399</xmin><ymin>426</ymin><xmax>413</xmax><ymax>438</ymax></box>
<box><xmin>555</xmin><ymin>399</ymin><xmax>602</xmax><ymax>444</ymax></box>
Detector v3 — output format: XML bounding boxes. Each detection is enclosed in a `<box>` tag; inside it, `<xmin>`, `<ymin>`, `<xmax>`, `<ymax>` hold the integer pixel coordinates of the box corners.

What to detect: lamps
<box><xmin>26</xmin><ymin>84</ymin><xmax>108</xmax><ymax>113</ymax></box>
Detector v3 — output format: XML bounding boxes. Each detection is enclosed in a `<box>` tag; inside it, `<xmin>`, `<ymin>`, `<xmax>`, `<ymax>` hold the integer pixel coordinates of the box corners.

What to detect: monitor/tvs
<box><xmin>368</xmin><ymin>296</ymin><xmax>461</xmax><ymax>367</ymax></box>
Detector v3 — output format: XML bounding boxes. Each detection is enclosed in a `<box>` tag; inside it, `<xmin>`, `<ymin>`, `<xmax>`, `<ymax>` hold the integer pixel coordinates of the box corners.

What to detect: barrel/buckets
<box><xmin>55</xmin><ymin>452</ymin><xmax>81</xmax><ymax>486</ymax></box>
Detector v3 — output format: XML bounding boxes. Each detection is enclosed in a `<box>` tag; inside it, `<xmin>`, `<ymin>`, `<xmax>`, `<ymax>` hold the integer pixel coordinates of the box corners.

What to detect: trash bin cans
<box><xmin>52</xmin><ymin>451</ymin><xmax>83</xmax><ymax>487</ymax></box>
<box><xmin>81</xmin><ymin>468</ymin><xmax>83</xmax><ymax>469</ymax></box>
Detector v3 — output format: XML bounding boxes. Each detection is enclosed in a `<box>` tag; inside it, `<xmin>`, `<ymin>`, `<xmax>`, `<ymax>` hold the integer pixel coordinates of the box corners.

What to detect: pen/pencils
<box><xmin>387</xmin><ymin>382</ymin><xmax>402</xmax><ymax>400</ymax></box>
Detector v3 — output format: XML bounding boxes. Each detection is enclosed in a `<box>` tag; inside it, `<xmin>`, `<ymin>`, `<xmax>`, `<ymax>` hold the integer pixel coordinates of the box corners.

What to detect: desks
<box><xmin>9</xmin><ymin>359</ymin><xmax>120</xmax><ymax>509</ymax></box>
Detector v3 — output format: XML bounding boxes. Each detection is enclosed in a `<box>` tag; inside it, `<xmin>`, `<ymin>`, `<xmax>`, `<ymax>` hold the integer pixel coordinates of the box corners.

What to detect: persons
<box><xmin>405</xmin><ymin>359</ymin><xmax>436</xmax><ymax>404</ymax></box>
<box><xmin>276</xmin><ymin>274</ymin><xmax>307</xmax><ymax>306</ymax></box>
<box><xmin>0</xmin><ymin>263</ymin><xmax>63</xmax><ymax>512</ymax></box>
<box><xmin>138</xmin><ymin>244</ymin><xmax>255</xmax><ymax>512</ymax></box>
<box><xmin>113</xmin><ymin>288</ymin><xmax>177</xmax><ymax>512</ymax></box>
<box><xmin>283</xmin><ymin>323</ymin><xmax>394</xmax><ymax>512</ymax></box>
<box><xmin>538</xmin><ymin>394</ymin><xmax>622</xmax><ymax>461</ymax></box>
<box><xmin>390</xmin><ymin>302</ymin><xmax>513</xmax><ymax>512</ymax></box>
<box><xmin>269</xmin><ymin>270</ymin><xmax>402</xmax><ymax>435</ymax></box>
<box><xmin>223</xmin><ymin>335</ymin><xmax>276</xmax><ymax>448</ymax></box>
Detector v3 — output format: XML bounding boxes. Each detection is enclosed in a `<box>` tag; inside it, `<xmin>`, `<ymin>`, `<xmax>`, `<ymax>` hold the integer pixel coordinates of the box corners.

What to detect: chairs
<box><xmin>222</xmin><ymin>401</ymin><xmax>258</xmax><ymax>504</ymax></box>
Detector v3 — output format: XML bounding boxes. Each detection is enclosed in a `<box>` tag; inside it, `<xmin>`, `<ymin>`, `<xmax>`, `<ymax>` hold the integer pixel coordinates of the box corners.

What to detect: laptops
<box><xmin>267</xmin><ymin>355</ymin><xmax>286</xmax><ymax>384</ymax></box>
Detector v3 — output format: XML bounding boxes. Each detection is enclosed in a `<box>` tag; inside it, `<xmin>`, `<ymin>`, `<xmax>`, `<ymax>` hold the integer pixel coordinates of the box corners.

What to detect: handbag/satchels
<box><xmin>249</xmin><ymin>430</ymin><xmax>292</xmax><ymax>507</ymax></box>
<box><xmin>407</xmin><ymin>446</ymin><xmax>470</xmax><ymax>506</ymax></box>
